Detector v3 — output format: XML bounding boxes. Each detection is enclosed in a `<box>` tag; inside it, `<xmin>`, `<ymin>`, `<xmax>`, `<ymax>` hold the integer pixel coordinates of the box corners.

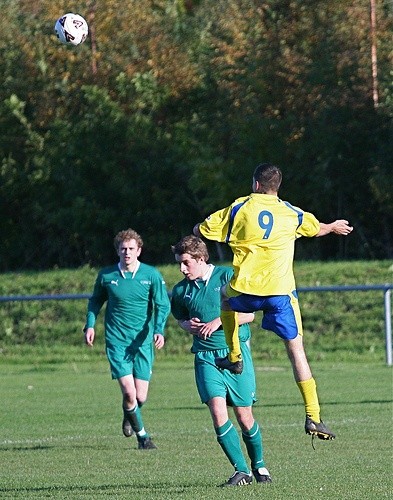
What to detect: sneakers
<box><xmin>221</xmin><ymin>471</ymin><xmax>253</xmax><ymax>487</ymax></box>
<box><xmin>215</xmin><ymin>354</ymin><xmax>243</xmax><ymax>374</ymax></box>
<box><xmin>305</xmin><ymin>417</ymin><xmax>335</xmax><ymax>440</ymax></box>
<box><xmin>122</xmin><ymin>414</ymin><xmax>134</xmax><ymax>437</ymax></box>
<box><xmin>137</xmin><ymin>437</ymin><xmax>157</xmax><ymax>449</ymax></box>
<box><xmin>252</xmin><ymin>468</ymin><xmax>271</xmax><ymax>484</ymax></box>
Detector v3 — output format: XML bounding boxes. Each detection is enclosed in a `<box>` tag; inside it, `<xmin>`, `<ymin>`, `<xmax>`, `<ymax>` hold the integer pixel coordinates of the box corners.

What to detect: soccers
<box><xmin>54</xmin><ymin>12</ymin><xmax>89</xmax><ymax>47</ymax></box>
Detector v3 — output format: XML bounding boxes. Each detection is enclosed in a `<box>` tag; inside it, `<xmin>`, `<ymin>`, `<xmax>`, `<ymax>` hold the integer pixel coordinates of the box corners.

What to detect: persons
<box><xmin>83</xmin><ymin>227</ymin><xmax>172</xmax><ymax>450</ymax></box>
<box><xmin>194</xmin><ymin>164</ymin><xmax>353</xmax><ymax>440</ymax></box>
<box><xmin>171</xmin><ymin>235</ymin><xmax>272</xmax><ymax>486</ymax></box>
